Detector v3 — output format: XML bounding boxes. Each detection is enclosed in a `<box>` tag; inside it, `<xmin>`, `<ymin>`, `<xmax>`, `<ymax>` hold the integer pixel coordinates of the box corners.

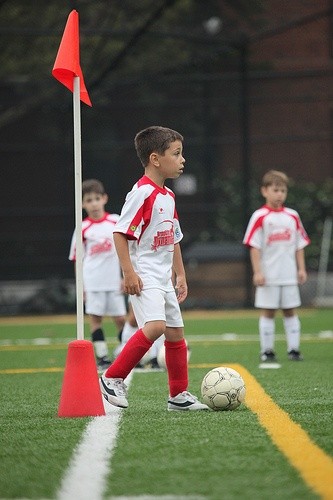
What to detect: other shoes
<box><xmin>288</xmin><ymin>349</ymin><xmax>304</xmax><ymax>361</ymax></box>
<box><xmin>258</xmin><ymin>350</ymin><xmax>280</xmax><ymax>369</ymax></box>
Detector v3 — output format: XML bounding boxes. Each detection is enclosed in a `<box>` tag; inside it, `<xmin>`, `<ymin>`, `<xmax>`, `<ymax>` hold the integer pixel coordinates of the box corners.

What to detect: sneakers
<box><xmin>167</xmin><ymin>390</ymin><xmax>210</xmax><ymax>411</ymax></box>
<box><xmin>96</xmin><ymin>370</ymin><xmax>130</xmax><ymax>408</ymax></box>
<box><xmin>92</xmin><ymin>340</ymin><xmax>113</xmax><ymax>370</ymax></box>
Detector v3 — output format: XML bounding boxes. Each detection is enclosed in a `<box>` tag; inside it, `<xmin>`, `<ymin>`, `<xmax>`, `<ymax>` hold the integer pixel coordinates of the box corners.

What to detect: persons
<box><xmin>98</xmin><ymin>125</ymin><xmax>210</xmax><ymax>412</ymax></box>
<box><xmin>242</xmin><ymin>170</ymin><xmax>311</xmax><ymax>369</ymax></box>
<box><xmin>67</xmin><ymin>179</ymin><xmax>129</xmax><ymax>368</ymax></box>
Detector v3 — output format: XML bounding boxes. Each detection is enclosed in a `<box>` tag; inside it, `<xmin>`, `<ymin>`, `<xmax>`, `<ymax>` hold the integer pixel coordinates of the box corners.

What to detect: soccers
<box><xmin>200</xmin><ymin>366</ymin><xmax>245</xmax><ymax>411</ymax></box>
